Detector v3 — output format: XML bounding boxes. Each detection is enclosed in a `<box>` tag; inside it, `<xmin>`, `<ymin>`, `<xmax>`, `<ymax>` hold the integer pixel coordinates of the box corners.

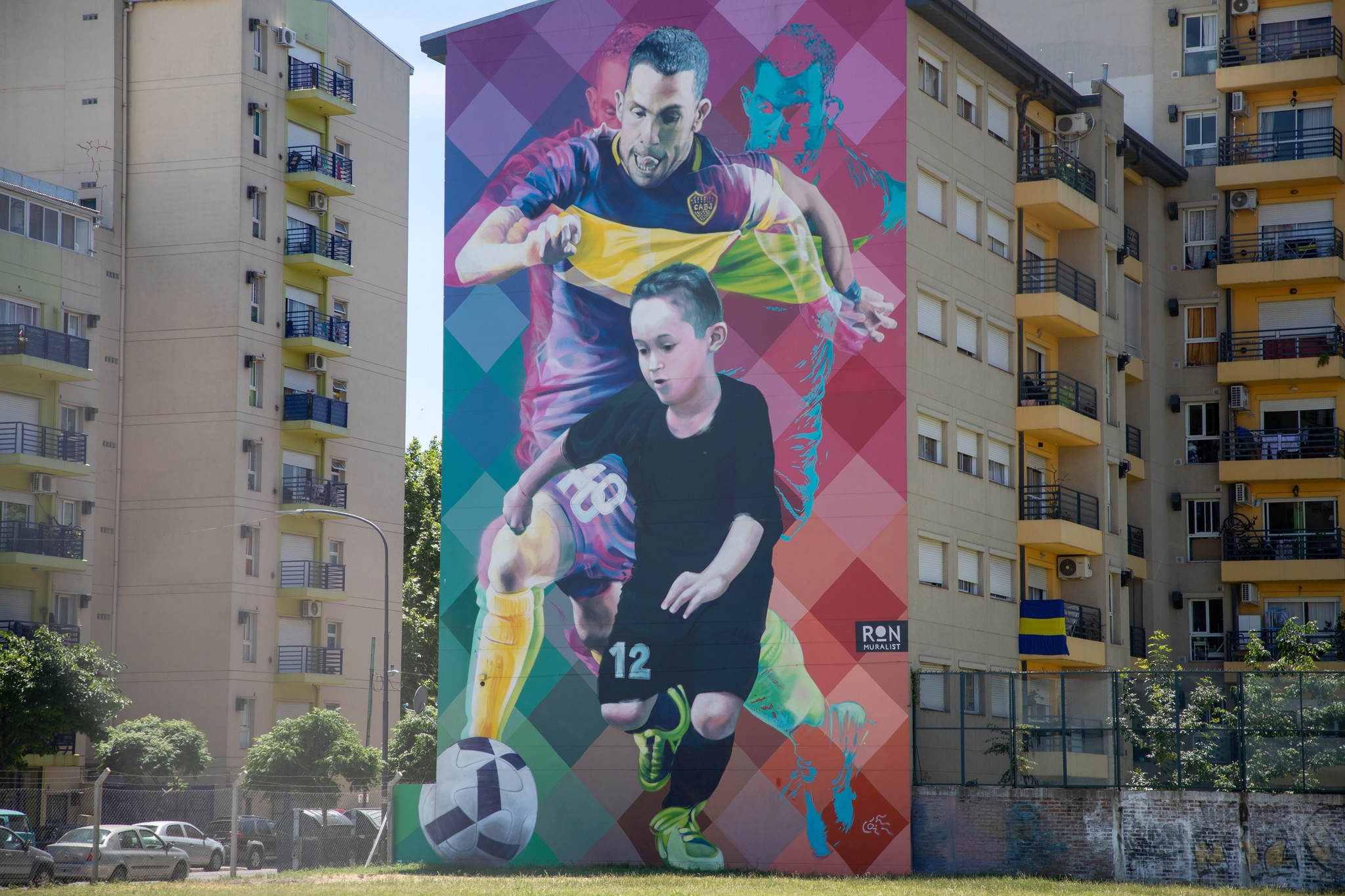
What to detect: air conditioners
<box><xmin>1242</xmin><ymin>583</ymin><xmax>1259</xmax><ymax>603</ymax></box>
<box><xmin>1231</xmin><ymin>0</ymin><xmax>1259</xmax><ymax>16</ymax></box>
<box><xmin>303</xmin><ymin>600</ymin><xmax>321</xmax><ymax>618</ymax></box>
<box><xmin>1236</xmin><ymin>483</ymin><xmax>1252</xmax><ymax>504</ymax></box>
<box><xmin>1057</xmin><ymin>556</ymin><xmax>1092</xmax><ymax>578</ymax></box>
<box><xmin>309</xmin><ymin>353</ymin><xmax>327</xmax><ymax>371</ymax></box>
<box><xmin>1230</xmin><ymin>189</ymin><xmax>1258</xmax><ymax>211</ymax></box>
<box><xmin>1231</xmin><ymin>386</ymin><xmax>1251</xmax><ymax>412</ymax></box>
<box><xmin>278</xmin><ymin>27</ymin><xmax>296</xmax><ymax>48</ymax></box>
<box><xmin>33</xmin><ymin>473</ymin><xmax>56</xmax><ymax>493</ymax></box>
<box><xmin>310</xmin><ymin>192</ymin><xmax>327</xmax><ymax>212</ymax></box>
<box><xmin>1056</xmin><ymin>113</ymin><xmax>1088</xmax><ymax>135</ymax></box>
<box><xmin>1232</xmin><ymin>91</ymin><xmax>1248</xmax><ymax>116</ymax></box>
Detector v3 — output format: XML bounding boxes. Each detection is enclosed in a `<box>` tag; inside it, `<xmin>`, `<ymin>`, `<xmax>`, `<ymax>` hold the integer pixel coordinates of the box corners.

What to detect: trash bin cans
<box><xmin>341</xmin><ymin>809</ymin><xmax>388</xmax><ymax>865</ymax></box>
<box><xmin>273</xmin><ymin>808</ymin><xmax>356</xmax><ymax>873</ymax></box>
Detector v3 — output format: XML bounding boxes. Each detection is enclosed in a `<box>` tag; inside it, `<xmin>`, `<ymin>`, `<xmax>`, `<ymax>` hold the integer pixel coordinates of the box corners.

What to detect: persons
<box><xmin>502</xmin><ymin>261</ymin><xmax>781</xmax><ymax>873</ymax></box>
<box><xmin>458</xmin><ymin>26</ymin><xmax>897</xmax><ymax>742</ymax></box>
<box><xmin>286</xmin><ymin>320</ymin><xmax>293</xmax><ymax>326</ymax></box>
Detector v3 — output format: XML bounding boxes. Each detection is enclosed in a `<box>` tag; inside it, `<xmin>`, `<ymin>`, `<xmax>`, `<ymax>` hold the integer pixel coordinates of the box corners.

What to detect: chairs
<box><xmin>120</xmin><ymin>836</ymin><xmax>138</xmax><ymax>849</ymax></box>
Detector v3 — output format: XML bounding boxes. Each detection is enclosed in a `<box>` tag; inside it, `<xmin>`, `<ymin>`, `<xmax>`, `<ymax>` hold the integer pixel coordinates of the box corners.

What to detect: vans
<box><xmin>0</xmin><ymin>809</ymin><xmax>36</xmax><ymax>850</ymax></box>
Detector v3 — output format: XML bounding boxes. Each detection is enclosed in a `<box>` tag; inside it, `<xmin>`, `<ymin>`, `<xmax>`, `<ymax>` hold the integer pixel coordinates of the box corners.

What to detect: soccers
<box><xmin>418</xmin><ymin>735</ymin><xmax>539</xmax><ymax>864</ymax></box>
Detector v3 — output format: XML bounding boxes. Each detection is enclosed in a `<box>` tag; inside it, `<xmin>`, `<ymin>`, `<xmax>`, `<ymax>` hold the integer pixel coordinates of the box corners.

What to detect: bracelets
<box><xmin>835</xmin><ymin>277</ymin><xmax>862</xmax><ymax>306</ymax></box>
<box><xmin>517</xmin><ymin>479</ymin><xmax>533</xmax><ymax>500</ymax></box>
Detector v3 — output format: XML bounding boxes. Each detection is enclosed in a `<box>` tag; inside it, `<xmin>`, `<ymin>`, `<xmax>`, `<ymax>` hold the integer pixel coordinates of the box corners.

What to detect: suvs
<box><xmin>203</xmin><ymin>815</ymin><xmax>277</xmax><ymax>871</ymax></box>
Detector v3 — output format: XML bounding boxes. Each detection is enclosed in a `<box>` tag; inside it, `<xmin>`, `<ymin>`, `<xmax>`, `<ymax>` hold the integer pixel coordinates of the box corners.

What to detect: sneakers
<box><xmin>633</xmin><ymin>685</ymin><xmax>693</xmax><ymax>795</ymax></box>
<box><xmin>647</xmin><ymin>798</ymin><xmax>724</xmax><ymax>874</ymax></box>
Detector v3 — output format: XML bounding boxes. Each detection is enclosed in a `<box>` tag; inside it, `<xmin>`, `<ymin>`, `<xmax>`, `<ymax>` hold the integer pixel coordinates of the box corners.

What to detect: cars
<box><xmin>44</xmin><ymin>824</ymin><xmax>191</xmax><ymax>884</ymax></box>
<box><xmin>132</xmin><ymin>820</ymin><xmax>226</xmax><ymax>872</ymax></box>
<box><xmin>0</xmin><ymin>825</ymin><xmax>55</xmax><ymax>888</ymax></box>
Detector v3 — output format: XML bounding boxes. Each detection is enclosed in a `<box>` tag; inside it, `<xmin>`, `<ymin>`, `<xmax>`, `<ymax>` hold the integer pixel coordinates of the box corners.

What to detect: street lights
<box><xmin>274</xmin><ymin>507</ymin><xmax>392</xmax><ymax>873</ymax></box>
